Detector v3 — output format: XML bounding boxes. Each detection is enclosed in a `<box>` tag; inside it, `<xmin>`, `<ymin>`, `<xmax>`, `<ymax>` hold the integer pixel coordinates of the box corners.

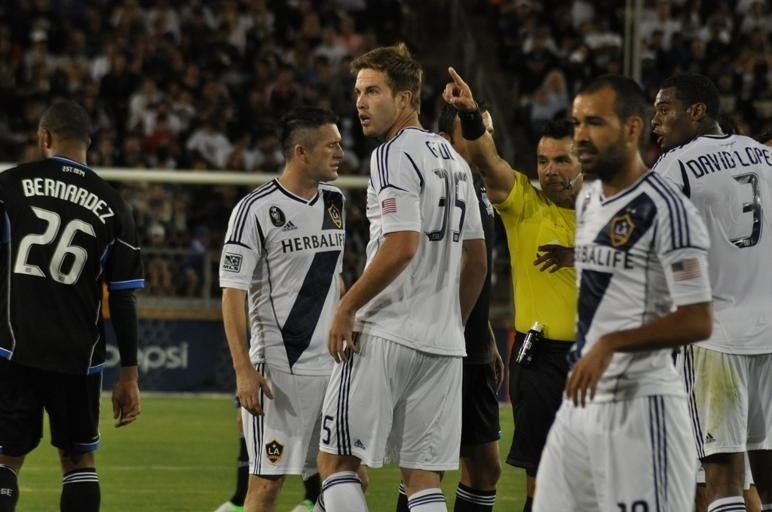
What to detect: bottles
<box><xmin>515</xmin><ymin>322</ymin><xmax>545</xmax><ymax>368</ymax></box>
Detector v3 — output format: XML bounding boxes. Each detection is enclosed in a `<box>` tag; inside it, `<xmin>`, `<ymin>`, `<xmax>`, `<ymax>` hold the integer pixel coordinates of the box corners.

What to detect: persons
<box><xmin>219</xmin><ymin>104</ymin><xmax>368</xmax><ymax>511</ymax></box>
<box><xmin>316</xmin><ymin>46</ymin><xmax>489</xmax><ymax>512</ymax></box>
<box><xmin>651</xmin><ymin>70</ymin><xmax>772</xmax><ymax>512</ymax></box>
<box><xmin>442</xmin><ymin>65</ymin><xmax>586</xmax><ymax>511</ymax></box>
<box><xmin>397</xmin><ymin>98</ymin><xmax>505</xmax><ymax>512</ymax></box>
<box><xmin>2</xmin><ymin>98</ymin><xmax>142</xmax><ymax>512</ymax></box>
<box><xmin>695</xmin><ymin>445</ymin><xmax>762</xmax><ymax>511</ymax></box>
<box><xmin>213</xmin><ymin>389</ymin><xmax>319</xmax><ymax>512</ymax></box>
<box><xmin>530</xmin><ymin>73</ymin><xmax>713</xmax><ymax>511</ymax></box>
<box><xmin>1</xmin><ymin>1</ymin><xmax>771</xmax><ymax>296</ymax></box>
